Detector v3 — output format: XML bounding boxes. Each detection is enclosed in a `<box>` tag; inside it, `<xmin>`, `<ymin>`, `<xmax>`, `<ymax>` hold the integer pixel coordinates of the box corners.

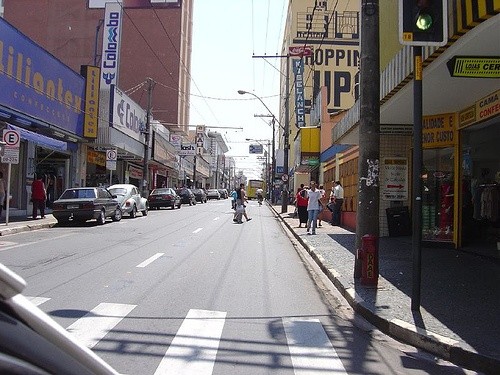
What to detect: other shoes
<box><xmin>332</xmin><ymin>224</ymin><xmax>339</xmax><ymax>226</ymax></box>
<box><xmin>331</xmin><ymin>223</ymin><xmax>336</xmax><ymax>225</ymax></box>
<box><xmin>42</xmin><ymin>216</ymin><xmax>46</xmax><ymax>219</ymax></box>
<box><xmin>247</xmin><ymin>218</ymin><xmax>252</xmax><ymax>221</ymax></box>
<box><xmin>319</xmin><ymin>225</ymin><xmax>322</xmax><ymax>226</ymax></box>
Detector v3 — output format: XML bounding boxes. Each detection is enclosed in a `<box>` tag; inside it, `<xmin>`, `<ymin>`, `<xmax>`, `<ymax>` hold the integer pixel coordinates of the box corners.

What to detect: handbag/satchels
<box><xmin>294</xmin><ymin>208</ymin><xmax>297</xmax><ymax>216</ymax></box>
<box><xmin>236</xmin><ymin>205</ymin><xmax>244</xmax><ymax>214</ymax></box>
<box><xmin>326</xmin><ymin>202</ymin><xmax>335</xmax><ymax>212</ymax></box>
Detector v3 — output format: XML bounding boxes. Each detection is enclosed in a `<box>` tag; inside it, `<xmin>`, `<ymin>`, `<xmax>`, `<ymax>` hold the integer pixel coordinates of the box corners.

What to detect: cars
<box><xmin>175</xmin><ymin>187</ymin><xmax>197</xmax><ymax>205</ymax></box>
<box><xmin>206</xmin><ymin>189</ymin><xmax>220</xmax><ymax>200</ymax></box>
<box><xmin>148</xmin><ymin>187</ymin><xmax>182</xmax><ymax>209</ymax></box>
<box><xmin>51</xmin><ymin>187</ymin><xmax>123</xmax><ymax>224</ymax></box>
<box><xmin>107</xmin><ymin>183</ymin><xmax>148</xmax><ymax>217</ymax></box>
<box><xmin>219</xmin><ymin>188</ymin><xmax>229</xmax><ymax>198</ymax></box>
<box><xmin>191</xmin><ymin>188</ymin><xmax>207</xmax><ymax>203</ymax></box>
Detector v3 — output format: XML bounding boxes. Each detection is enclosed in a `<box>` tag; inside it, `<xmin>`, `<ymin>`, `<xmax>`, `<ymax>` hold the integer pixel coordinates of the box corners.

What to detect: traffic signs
<box><xmin>277</xmin><ymin>165</ymin><xmax>284</xmax><ymax>172</ymax></box>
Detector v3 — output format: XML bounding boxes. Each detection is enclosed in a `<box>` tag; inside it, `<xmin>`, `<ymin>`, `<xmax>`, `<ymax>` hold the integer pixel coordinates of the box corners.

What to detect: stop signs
<box><xmin>281</xmin><ymin>174</ymin><xmax>289</xmax><ymax>182</ymax></box>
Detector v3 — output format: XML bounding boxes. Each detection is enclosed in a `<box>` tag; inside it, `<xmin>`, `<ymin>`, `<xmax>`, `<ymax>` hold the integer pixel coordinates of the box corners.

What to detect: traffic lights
<box><xmin>398</xmin><ymin>0</ymin><xmax>449</xmax><ymax>45</ymax></box>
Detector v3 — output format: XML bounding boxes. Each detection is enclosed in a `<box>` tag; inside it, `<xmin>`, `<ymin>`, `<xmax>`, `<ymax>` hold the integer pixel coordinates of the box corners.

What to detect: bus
<box><xmin>247</xmin><ymin>179</ymin><xmax>266</xmax><ymax>200</ymax></box>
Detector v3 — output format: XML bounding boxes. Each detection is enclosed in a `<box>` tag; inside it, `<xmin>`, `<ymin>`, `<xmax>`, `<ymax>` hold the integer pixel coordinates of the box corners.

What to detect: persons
<box><xmin>30</xmin><ymin>175</ymin><xmax>48</xmax><ymax>220</ymax></box>
<box><xmin>230</xmin><ymin>181</ymin><xmax>343</xmax><ymax>228</ymax></box>
<box><xmin>305</xmin><ymin>181</ymin><xmax>325</xmax><ymax>235</ymax></box>
<box><xmin>0</xmin><ymin>172</ymin><xmax>7</xmax><ymax>220</ymax></box>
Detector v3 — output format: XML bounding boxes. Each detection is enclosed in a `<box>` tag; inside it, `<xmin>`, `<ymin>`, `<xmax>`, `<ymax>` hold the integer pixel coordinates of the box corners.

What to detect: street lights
<box><xmin>244</xmin><ymin>138</ymin><xmax>276</xmax><ymax>204</ymax></box>
<box><xmin>238</xmin><ymin>90</ymin><xmax>289</xmax><ymax>213</ymax></box>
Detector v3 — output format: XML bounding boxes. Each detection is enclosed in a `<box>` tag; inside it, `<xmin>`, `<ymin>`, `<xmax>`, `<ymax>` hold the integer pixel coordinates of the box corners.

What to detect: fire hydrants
<box><xmin>356</xmin><ymin>233</ymin><xmax>378</xmax><ymax>285</ymax></box>
